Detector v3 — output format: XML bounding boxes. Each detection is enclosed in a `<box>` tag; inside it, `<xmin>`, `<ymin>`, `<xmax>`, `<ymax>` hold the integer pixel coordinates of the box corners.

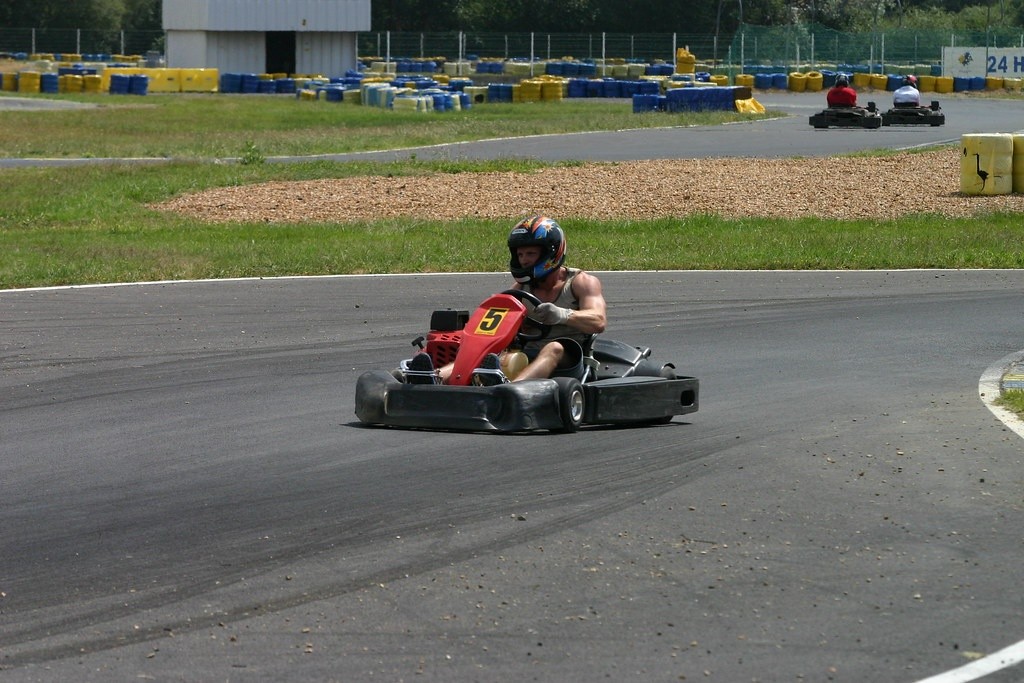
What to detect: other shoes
<box><xmin>409</xmin><ymin>352</ymin><xmax>435</xmax><ymax>384</ymax></box>
<box><xmin>478</xmin><ymin>353</ymin><xmax>511</xmax><ymax>386</ymax></box>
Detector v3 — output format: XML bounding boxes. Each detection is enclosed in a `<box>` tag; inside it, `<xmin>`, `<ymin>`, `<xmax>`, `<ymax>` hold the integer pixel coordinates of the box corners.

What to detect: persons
<box><xmin>892</xmin><ymin>75</ymin><xmax>919</xmax><ymax>106</ymax></box>
<box><xmin>827</xmin><ymin>74</ymin><xmax>858</xmax><ymax>107</ymax></box>
<box><xmin>409</xmin><ymin>216</ymin><xmax>607</xmax><ymax>384</ymax></box>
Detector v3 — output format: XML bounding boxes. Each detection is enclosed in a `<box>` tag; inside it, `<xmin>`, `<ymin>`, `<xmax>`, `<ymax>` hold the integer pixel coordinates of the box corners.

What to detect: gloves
<box><xmin>532</xmin><ymin>302</ymin><xmax>573</xmax><ymax>325</ymax></box>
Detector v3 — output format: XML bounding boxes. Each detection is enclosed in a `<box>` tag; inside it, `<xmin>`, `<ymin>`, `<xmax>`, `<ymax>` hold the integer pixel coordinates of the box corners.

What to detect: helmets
<box><xmin>835</xmin><ymin>73</ymin><xmax>849</xmax><ymax>87</ymax></box>
<box><xmin>903</xmin><ymin>75</ymin><xmax>917</xmax><ymax>88</ymax></box>
<box><xmin>507</xmin><ymin>216</ymin><xmax>566</xmax><ymax>285</ymax></box>
